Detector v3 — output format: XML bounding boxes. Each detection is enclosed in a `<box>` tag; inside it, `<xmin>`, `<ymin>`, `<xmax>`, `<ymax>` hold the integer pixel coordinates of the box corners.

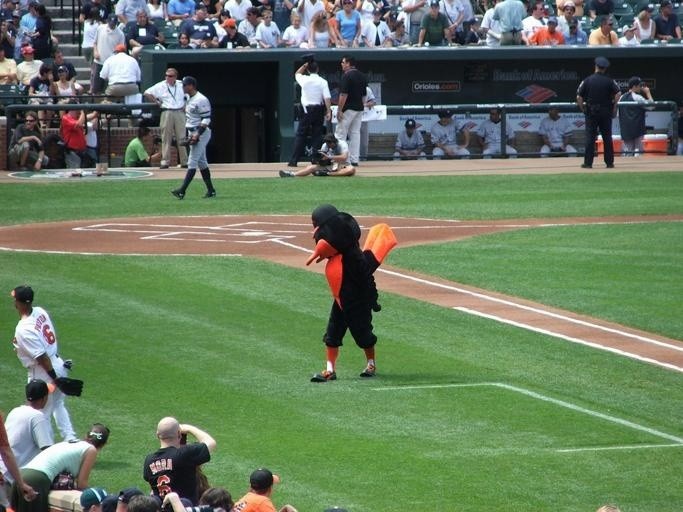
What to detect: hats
<box><xmin>439</xmin><ymin>110</ymin><xmax>454</xmax><ymax>118</ymax></box>
<box><xmin>547</xmin><ymin>16</ymin><xmax>557</xmax><ymax>24</ymax></box>
<box><xmin>250</xmin><ymin>468</ymin><xmax>279</xmax><ymax>489</ymax></box>
<box><xmin>58</xmin><ymin>66</ymin><xmax>68</xmax><ymax>72</ymax></box>
<box><xmin>26</xmin><ymin>380</ymin><xmax>55</xmax><ymax>400</ymax></box>
<box><xmin>629</xmin><ymin>76</ymin><xmax>643</xmax><ymax>87</ymax></box>
<box><xmin>222</xmin><ymin>19</ymin><xmax>234</xmax><ymax>27</ymax></box>
<box><xmin>21</xmin><ymin>47</ymin><xmax>34</xmax><ymax>55</ymax></box>
<box><xmin>118</xmin><ymin>488</ymin><xmax>143</xmax><ymax>503</ymax></box>
<box><xmin>623</xmin><ymin>25</ymin><xmax>636</xmax><ymax>34</ymax></box>
<box><xmin>80</xmin><ymin>488</ymin><xmax>107</xmax><ymax>506</ymax></box>
<box><xmin>115</xmin><ymin>45</ymin><xmax>125</xmax><ymax>52</ymax></box>
<box><xmin>181</xmin><ymin>77</ymin><xmax>197</xmax><ymax>85</ymax></box>
<box><xmin>595</xmin><ymin>57</ymin><xmax>609</xmax><ymax>68</ymax></box>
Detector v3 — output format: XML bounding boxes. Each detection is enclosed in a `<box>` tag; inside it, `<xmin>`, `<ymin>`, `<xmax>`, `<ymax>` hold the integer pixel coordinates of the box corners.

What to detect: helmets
<box><xmin>405</xmin><ymin>120</ymin><xmax>416</xmax><ymax>129</ymax></box>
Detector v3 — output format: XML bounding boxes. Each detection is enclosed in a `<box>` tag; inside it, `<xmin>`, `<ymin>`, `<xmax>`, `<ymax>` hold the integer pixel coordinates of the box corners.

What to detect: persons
<box><xmin>0</xmin><ymin>380</ymin><xmax>298</xmax><ymax>512</ymax></box>
<box><xmin>92</xmin><ymin>1</ymin><xmax>683</xmax><ymax>105</ymax></box>
<box><xmin>279</xmin><ymin>54</ymin><xmax>376</xmax><ymax>177</ymax></box>
<box><xmin>596</xmin><ymin>505</ymin><xmax>620</xmax><ymax>512</ymax></box>
<box><xmin>143</xmin><ymin>68</ymin><xmax>216</xmax><ymax>199</ymax></box>
<box><xmin>125</xmin><ymin>127</ymin><xmax>161</xmax><ymax>166</ymax></box>
<box><xmin>11</xmin><ymin>285</ymin><xmax>77</xmax><ymax>444</ymax></box>
<box><xmin>393</xmin><ymin>109</ymin><xmax>517</xmax><ymax>161</ymax></box>
<box><xmin>537</xmin><ymin>56</ymin><xmax>683</xmax><ymax>168</ymax></box>
<box><xmin>1</xmin><ymin>2</ymin><xmax>111</xmax><ymax>172</ymax></box>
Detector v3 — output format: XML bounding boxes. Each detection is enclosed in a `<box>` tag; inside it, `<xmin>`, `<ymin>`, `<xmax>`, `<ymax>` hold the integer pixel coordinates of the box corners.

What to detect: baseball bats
<box><xmin>72</xmin><ymin>171</ymin><xmax>123</xmax><ymax>177</ymax></box>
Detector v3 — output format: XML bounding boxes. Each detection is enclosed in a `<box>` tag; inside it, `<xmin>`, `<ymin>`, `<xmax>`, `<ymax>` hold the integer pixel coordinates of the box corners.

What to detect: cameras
<box><xmin>179</xmin><ymin>433</ymin><xmax>187</xmax><ymax>445</ymax></box>
<box><xmin>320</xmin><ymin>12</ymin><xmax>327</xmax><ymax>24</ymax></box>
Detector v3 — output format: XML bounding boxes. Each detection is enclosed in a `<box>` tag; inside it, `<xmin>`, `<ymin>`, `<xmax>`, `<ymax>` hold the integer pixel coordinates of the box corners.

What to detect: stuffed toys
<box><xmin>305</xmin><ymin>204</ymin><xmax>397</xmax><ymax>383</ymax></box>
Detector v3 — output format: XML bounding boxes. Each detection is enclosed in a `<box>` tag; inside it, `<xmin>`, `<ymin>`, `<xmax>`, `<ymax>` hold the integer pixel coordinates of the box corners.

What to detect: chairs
<box><xmin>0</xmin><ymin>83</ymin><xmax>30</xmax><ymax>118</ymax></box>
<box><xmin>104</xmin><ymin>0</ymin><xmax>682</xmax><ymax>51</ymax></box>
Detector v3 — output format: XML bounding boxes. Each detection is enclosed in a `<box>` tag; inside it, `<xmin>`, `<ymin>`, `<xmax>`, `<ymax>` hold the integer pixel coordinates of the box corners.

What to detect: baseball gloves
<box><xmin>55</xmin><ymin>377</ymin><xmax>83</xmax><ymax>396</ymax></box>
<box><xmin>178</xmin><ymin>134</ymin><xmax>199</xmax><ymax>146</ymax></box>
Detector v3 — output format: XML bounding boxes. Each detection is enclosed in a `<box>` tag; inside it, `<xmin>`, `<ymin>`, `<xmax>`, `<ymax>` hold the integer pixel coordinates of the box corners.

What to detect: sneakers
<box><xmin>280</xmin><ymin>171</ymin><xmax>291</xmax><ymax>176</ymax></box>
<box><xmin>313</xmin><ymin>170</ymin><xmax>327</xmax><ymax>176</ymax></box>
<box><xmin>205</xmin><ymin>189</ymin><xmax>216</xmax><ymax>197</ymax></box>
<box><xmin>172</xmin><ymin>190</ymin><xmax>185</xmax><ymax>199</ymax></box>
<box><xmin>11</xmin><ymin>286</ymin><xmax>33</xmax><ymax>303</ymax></box>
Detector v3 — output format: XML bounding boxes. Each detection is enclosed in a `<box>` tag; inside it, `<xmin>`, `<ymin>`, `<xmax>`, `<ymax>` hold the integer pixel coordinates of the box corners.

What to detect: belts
<box><xmin>188</xmin><ymin>127</ymin><xmax>199</xmax><ymax>131</ymax></box>
<box><xmin>114</xmin><ymin>82</ymin><xmax>134</xmax><ymax>84</ymax></box>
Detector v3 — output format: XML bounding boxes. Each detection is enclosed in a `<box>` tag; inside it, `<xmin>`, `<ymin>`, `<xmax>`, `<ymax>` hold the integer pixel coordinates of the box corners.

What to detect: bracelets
<box><xmin>47</xmin><ymin>368</ymin><xmax>56</xmax><ymax>379</ymax></box>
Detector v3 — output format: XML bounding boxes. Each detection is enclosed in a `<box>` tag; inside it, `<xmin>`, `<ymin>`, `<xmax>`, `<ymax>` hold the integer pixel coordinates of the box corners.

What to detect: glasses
<box><xmin>165</xmin><ymin>74</ymin><xmax>172</xmax><ymax>77</ymax></box>
<box><xmin>25</xmin><ymin>120</ymin><xmax>35</xmax><ymax>122</ymax></box>
